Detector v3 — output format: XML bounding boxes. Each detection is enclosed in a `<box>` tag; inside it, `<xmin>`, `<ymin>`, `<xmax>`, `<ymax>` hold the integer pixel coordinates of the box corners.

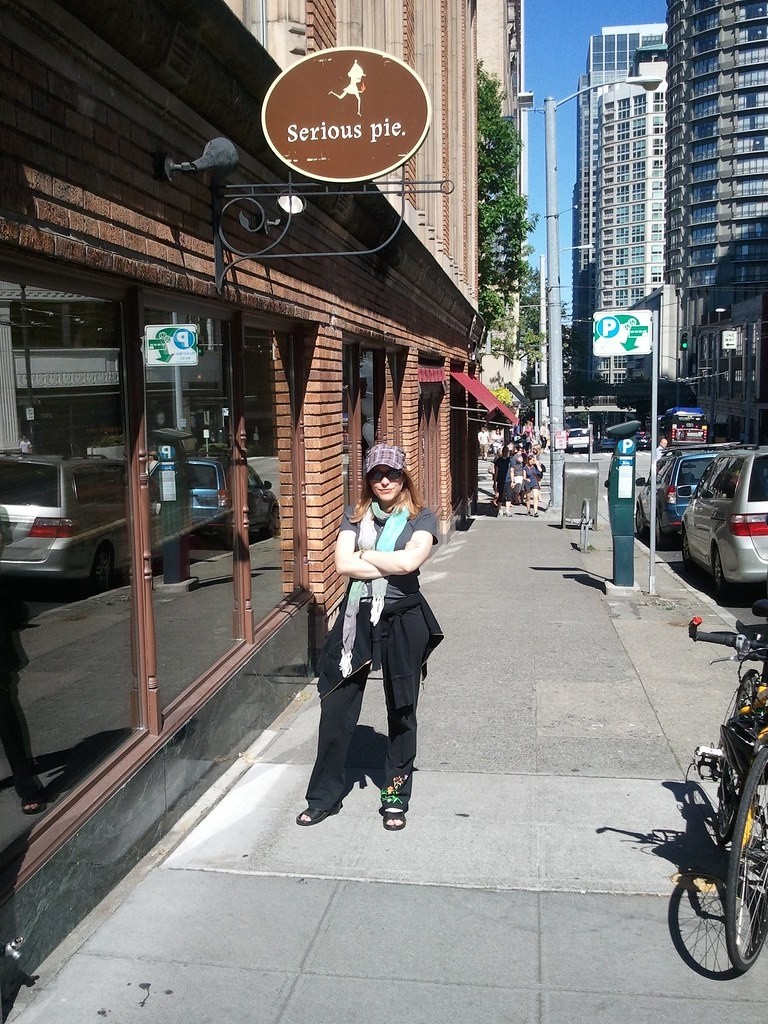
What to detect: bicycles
<box><xmin>687</xmin><ymin>597</ymin><xmax>768</xmax><ymax>974</ymax></box>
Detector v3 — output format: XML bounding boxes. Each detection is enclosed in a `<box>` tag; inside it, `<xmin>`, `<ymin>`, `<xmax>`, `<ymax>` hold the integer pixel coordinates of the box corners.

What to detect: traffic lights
<box><xmin>197</xmin><ymin>336</ymin><xmax>206</xmax><ymax>356</ymax></box>
<box><xmin>680</xmin><ymin>328</ymin><xmax>690</xmax><ymax>353</ymax></box>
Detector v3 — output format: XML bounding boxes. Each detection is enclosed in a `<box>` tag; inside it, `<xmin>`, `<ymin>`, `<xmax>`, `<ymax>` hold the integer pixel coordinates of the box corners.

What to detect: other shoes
<box><xmin>534</xmin><ymin>513</ymin><xmax>539</xmax><ymax>517</ymax></box>
<box><xmin>499</xmin><ymin>510</ymin><xmax>504</xmax><ymax>516</ymax></box>
<box><xmin>505</xmin><ymin>511</ymin><xmax>512</xmax><ymax>517</ymax></box>
<box><xmin>527</xmin><ymin>512</ymin><xmax>533</xmax><ymax>516</ymax></box>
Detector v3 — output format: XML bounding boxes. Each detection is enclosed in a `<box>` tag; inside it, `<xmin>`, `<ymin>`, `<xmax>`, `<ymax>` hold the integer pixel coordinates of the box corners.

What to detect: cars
<box><xmin>563</xmin><ymin>406</ymin><xmax>710</xmax><ymax>455</ymax></box>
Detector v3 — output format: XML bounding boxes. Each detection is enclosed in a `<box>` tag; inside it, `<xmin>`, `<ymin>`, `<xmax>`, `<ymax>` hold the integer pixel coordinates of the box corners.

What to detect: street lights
<box><xmin>539</xmin><ymin>243</ymin><xmax>595</xmax><ymax>428</ymax></box>
<box><xmin>544</xmin><ymin>76</ymin><xmax>665</xmax><ymax>516</ymax></box>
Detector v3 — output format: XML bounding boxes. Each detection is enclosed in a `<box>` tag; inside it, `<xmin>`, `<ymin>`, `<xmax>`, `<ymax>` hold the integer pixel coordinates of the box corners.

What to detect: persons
<box><xmin>543</xmin><ymin>423</ymin><xmax>550</xmax><ymax>452</ymax></box>
<box><xmin>512</xmin><ymin>420</ymin><xmax>548</xmax><ymax>453</ymax></box>
<box><xmin>656</xmin><ymin>439</ymin><xmax>667</xmax><ymax>463</ymax></box>
<box><xmin>491</xmin><ymin>445</ymin><xmax>542</xmax><ymax>517</ymax></box>
<box><xmin>296</xmin><ymin>441</ymin><xmax>440</xmax><ymax>832</ymax></box>
<box><xmin>478</xmin><ymin>426</ymin><xmax>504</xmax><ymax>461</ymax></box>
<box><xmin>566</xmin><ymin>428</ymin><xmax>570</xmax><ymax>453</ymax></box>
<box><xmin>19</xmin><ymin>437</ymin><xmax>33</xmax><ymax>454</ymax></box>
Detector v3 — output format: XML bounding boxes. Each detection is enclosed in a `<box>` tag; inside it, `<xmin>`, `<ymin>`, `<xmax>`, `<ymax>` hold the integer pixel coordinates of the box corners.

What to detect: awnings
<box><xmin>449</xmin><ymin>369</ymin><xmax>498</xmax><ymax>423</ymax></box>
<box><xmin>505</xmin><ymin>383</ymin><xmax>529</xmax><ymax>406</ymax></box>
<box><xmin>469</xmin><ymin>374</ymin><xmax>520</xmax><ymax>427</ymax></box>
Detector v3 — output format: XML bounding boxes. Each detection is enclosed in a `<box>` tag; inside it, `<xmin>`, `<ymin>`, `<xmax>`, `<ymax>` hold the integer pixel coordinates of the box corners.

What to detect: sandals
<box><xmin>297</xmin><ymin>802</ymin><xmax>340</xmax><ymax>826</ymax></box>
<box><xmin>383</xmin><ymin>809</ymin><xmax>406</xmax><ymax>831</ymax></box>
<box><xmin>21</xmin><ymin>778</ymin><xmax>47</xmax><ymax>814</ymax></box>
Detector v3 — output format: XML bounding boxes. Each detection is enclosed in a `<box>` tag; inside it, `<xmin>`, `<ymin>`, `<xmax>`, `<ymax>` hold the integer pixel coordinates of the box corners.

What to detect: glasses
<box><xmin>369</xmin><ymin>468</ymin><xmax>403</xmax><ymax>481</ymax></box>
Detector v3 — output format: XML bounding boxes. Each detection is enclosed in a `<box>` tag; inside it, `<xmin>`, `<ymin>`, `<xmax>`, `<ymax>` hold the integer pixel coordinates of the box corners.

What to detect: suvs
<box><xmin>634</xmin><ymin>439</ymin><xmax>741</xmax><ymax>551</ymax></box>
<box><xmin>149</xmin><ymin>452</ymin><xmax>280</xmax><ymax>539</ymax></box>
<box><xmin>0</xmin><ymin>448</ymin><xmax>165</xmax><ymax>597</ymax></box>
<box><xmin>675</xmin><ymin>442</ymin><xmax>768</xmax><ymax>600</ymax></box>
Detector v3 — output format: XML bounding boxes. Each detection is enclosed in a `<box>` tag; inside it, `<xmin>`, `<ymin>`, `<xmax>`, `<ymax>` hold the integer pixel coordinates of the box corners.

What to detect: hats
<box><xmin>366</xmin><ymin>443</ymin><xmax>406</xmax><ymax>473</ymax></box>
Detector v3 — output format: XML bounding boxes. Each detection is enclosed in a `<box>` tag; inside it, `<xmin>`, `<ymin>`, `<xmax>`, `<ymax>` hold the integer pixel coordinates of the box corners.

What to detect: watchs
<box><xmin>359</xmin><ymin>548</ymin><xmax>369</xmax><ymax>558</ymax></box>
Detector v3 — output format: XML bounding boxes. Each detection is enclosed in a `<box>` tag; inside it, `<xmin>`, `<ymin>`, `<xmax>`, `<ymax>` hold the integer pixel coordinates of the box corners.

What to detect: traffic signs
<box><xmin>721</xmin><ymin>330</ymin><xmax>738</xmax><ymax>350</ymax></box>
